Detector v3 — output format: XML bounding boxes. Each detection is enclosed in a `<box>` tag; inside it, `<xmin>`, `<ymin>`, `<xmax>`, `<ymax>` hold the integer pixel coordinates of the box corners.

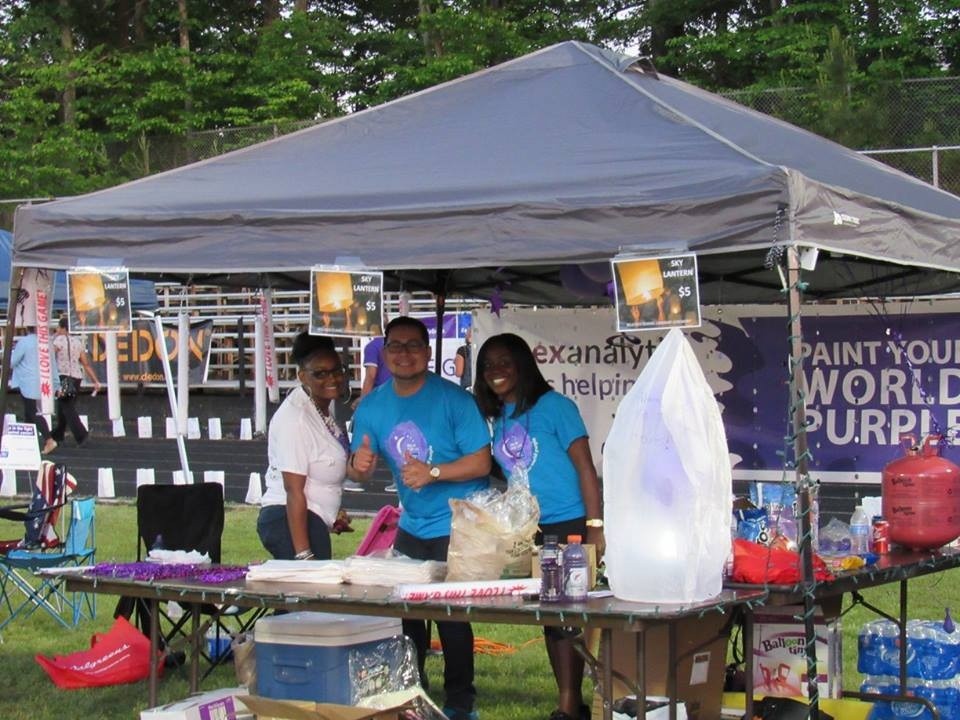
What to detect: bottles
<box><xmin>858</xmin><ymin>618</ymin><xmax>960</xmax><ymax>720</ymax></box>
<box><xmin>850</xmin><ymin>504</ymin><xmax>868</xmax><ymax>552</ymax></box>
<box><xmin>562</xmin><ymin>533</ymin><xmax>589</xmax><ymax>603</ymax></box>
<box><xmin>537</xmin><ymin>534</ymin><xmax>562</xmax><ymax>603</ymax></box>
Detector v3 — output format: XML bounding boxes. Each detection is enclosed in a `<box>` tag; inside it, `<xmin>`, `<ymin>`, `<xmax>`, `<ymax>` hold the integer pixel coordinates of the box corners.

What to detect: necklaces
<box><xmin>499</xmin><ymin>401</ymin><xmax>529</xmax><ymax>459</ymax></box>
<box><xmin>310</xmin><ymin>397</ymin><xmax>349</xmax><ymax>450</ymax></box>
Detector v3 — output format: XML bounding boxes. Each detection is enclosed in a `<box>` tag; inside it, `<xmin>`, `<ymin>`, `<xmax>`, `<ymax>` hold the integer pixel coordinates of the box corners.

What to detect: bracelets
<box><xmin>350</xmin><ymin>453</ymin><xmax>356</xmax><ymax>469</ymax></box>
<box><xmin>295</xmin><ymin>550</ymin><xmax>315</xmax><ymax>561</ymax></box>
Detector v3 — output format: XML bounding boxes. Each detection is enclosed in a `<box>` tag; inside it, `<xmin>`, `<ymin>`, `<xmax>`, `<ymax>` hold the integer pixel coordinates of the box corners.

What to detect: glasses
<box><xmin>303</xmin><ymin>365</ymin><xmax>347</xmax><ymax>379</ymax></box>
<box><xmin>385</xmin><ymin>342</ymin><xmax>427</xmax><ymax>354</ymax></box>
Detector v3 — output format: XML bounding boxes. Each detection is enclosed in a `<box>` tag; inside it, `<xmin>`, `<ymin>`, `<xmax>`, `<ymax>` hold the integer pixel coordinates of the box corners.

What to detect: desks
<box><xmin>720</xmin><ymin>536</ymin><xmax>960</xmax><ymax>720</ymax></box>
<box><xmin>36</xmin><ymin>560</ymin><xmax>770</xmax><ymax>720</ymax></box>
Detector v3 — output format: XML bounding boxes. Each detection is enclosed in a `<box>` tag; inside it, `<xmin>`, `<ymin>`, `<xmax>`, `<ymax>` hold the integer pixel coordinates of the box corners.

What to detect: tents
<box><xmin>12</xmin><ymin>40</ymin><xmax>960</xmax><ymax>720</ymax></box>
<box><xmin>0</xmin><ymin>225</ymin><xmax>193</xmax><ymax>489</ymax></box>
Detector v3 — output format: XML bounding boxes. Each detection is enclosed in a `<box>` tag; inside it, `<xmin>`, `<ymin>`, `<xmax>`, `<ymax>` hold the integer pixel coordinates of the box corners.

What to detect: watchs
<box><xmin>585</xmin><ymin>518</ymin><xmax>605</xmax><ymax>527</ymax></box>
<box><xmin>429</xmin><ymin>463</ymin><xmax>439</xmax><ymax>481</ymax></box>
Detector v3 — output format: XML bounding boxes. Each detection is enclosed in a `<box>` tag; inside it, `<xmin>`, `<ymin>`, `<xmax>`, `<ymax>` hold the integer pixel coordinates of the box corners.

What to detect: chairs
<box><xmin>1</xmin><ymin>462</ymin><xmax>67</xmax><ymax>616</ymax></box>
<box><xmin>0</xmin><ymin>494</ymin><xmax>97</xmax><ymax>632</ymax></box>
<box><xmin>135</xmin><ymin>481</ymin><xmax>270</xmax><ymax>685</ymax></box>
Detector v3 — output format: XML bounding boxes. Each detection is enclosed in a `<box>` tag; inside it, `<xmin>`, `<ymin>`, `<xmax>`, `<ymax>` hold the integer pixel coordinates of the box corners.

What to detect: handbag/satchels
<box><xmin>444</xmin><ymin>489</ymin><xmax>542</xmax><ymax>582</ymax></box>
<box><xmin>231</xmin><ymin>631</ymin><xmax>256</xmax><ymax>689</ymax></box>
<box><xmin>54</xmin><ymin>377</ymin><xmax>78</xmax><ymax>400</ymax></box>
<box><xmin>33</xmin><ymin>616</ymin><xmax>166</xmax><ymax>690</ymax></box>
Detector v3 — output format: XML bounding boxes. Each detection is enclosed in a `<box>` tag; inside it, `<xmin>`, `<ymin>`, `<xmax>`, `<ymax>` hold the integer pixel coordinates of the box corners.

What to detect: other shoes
<box><xmin>77</xmin><ymin>431</ymin><xmax>89</xmax><ymax>449</ymax></box>
<box><xmin>41</xmin><ymin>439</ymin><xmax>57</xmax><ymax>455</ymax></box>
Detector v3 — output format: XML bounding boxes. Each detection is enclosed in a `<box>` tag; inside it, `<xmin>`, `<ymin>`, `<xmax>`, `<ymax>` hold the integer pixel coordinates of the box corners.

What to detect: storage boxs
<box><xmin>140</xmin><ymin>687</ymin><xmax>255</xmax><ymax>720</ymax></box>
<box><xmin>749</xmin><ymin>592</ymin><xmax>845</xmax><ymax>701</ymax></box>
<box><xmin>254</xmin><ymin>609</ymin><xmax>404</xmax><ymax>708</ymax></box>
<box><xmin>233</xmin><ymin>686</ymin><xmax>423</xmax><ymax>720</ymax></box>
<box><xmin>591</xmin><ymin>606</ymin><xmax>735</xmax><ymax>720</ymax></box>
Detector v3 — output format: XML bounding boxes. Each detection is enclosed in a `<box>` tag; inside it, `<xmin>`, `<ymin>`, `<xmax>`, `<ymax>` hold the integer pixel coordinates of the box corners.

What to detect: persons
<box><xmin>343</xmin><ymin>315</ymin><xmax>400</xmax><ymax>494</ymax></box>
<box><xmin>346</xmin><ymin>314</ymin><xmax>493</xmax><ymax>720</ymax></box>
<box><xmin>50</xmin><ymin>318</ymin><xmax>102</xmax><ymax>447</ymax></box>
<box><xmin>7</xmin><ymin>325</ymin><xmax>59</xmax><ymax>454</ymax></box>
<box><xmin>469</xmin><ymin>332</ymin><xmax>606</xmax><ymax>720</ymax></box>
<box><xmin>453</xmin><ymin>322</ymin><xmax>470</xmax><ymax>390</ymax></box>
<box><xmin>256</xmin><ymin>331</ymin><xmax>354</xmax><ymax>561</ymax></box>
